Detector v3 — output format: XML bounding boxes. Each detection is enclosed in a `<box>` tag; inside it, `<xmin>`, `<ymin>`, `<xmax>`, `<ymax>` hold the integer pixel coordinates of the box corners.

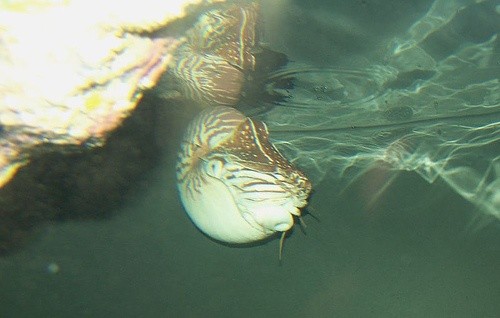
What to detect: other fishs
<box><xmin>142</xmin><ymin>0</ymin><xmax>260</xmax><ymax>108</ymax></box>
<box><xmin>174</xmin><ymin>105</ymin><xmax>312</xmax><ymax>260</ymax></box>
